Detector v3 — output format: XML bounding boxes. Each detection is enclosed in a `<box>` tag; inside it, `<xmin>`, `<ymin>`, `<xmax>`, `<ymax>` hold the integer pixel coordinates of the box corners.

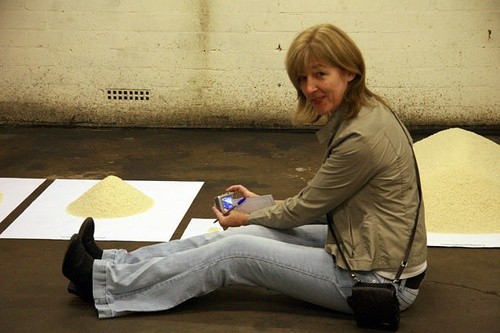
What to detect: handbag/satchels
<box><xmin>347</xmin><ymin>282</ymin><xmax>400</xmax><ymax>333</ymax></box>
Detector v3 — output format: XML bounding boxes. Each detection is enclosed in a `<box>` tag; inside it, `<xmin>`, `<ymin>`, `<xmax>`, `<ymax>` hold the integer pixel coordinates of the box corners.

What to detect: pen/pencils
<box><xmin>214</xmin><ymin>197</ymin><xmax>246</xmax><ymax>223</ymax></box>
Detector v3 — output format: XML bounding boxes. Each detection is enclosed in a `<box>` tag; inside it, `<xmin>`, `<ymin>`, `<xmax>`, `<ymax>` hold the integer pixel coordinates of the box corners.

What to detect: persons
<box><xmin>61</xmin><ymin>23</ymin><xmax>427</xmax><ymax>320</ymax></box>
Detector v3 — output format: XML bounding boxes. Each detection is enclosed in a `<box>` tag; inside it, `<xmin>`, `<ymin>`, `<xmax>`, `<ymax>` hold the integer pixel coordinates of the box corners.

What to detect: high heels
<box><xmin>62</xmin><ymin>233</ymin><xmax>94</xmax><ymax>303</ymax></box>
<box><xmin>78</xmin><ymin>216</ymin><xmax>103</xmax><ymax>260</ymax></box>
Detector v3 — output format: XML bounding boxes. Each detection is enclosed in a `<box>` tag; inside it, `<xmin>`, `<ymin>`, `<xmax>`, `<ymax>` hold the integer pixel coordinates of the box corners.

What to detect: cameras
<box><xmin>215</xmin><ymin>191</ymin><xmax>234</xmax><ymax>213</ymax></box>
